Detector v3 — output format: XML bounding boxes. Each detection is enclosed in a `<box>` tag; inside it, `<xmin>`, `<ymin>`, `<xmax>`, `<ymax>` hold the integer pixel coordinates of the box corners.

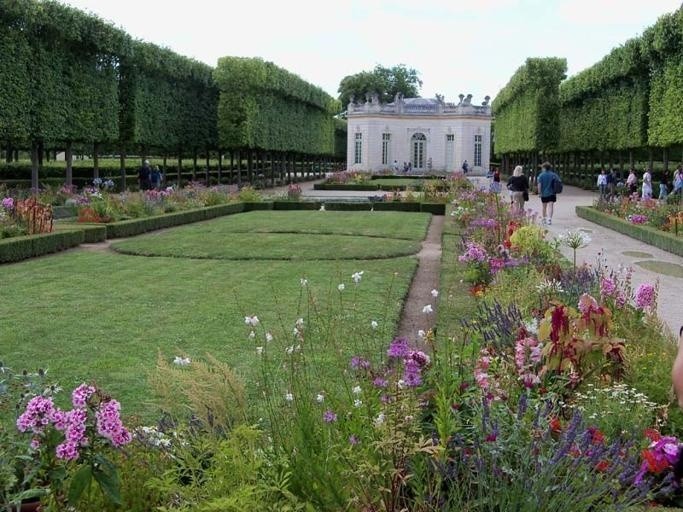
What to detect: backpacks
<box><xmin>551</xmin><ymin>172</ymin><xmax>563</xmax><ymax>194</ymax></box>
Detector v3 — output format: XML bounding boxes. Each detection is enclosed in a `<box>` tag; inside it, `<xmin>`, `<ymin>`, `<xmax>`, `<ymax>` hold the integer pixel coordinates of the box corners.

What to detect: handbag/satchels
<box><xmin>523</xmin><ymin>190</ymin><xmax>529</xmax><ymax>201</ymax></box>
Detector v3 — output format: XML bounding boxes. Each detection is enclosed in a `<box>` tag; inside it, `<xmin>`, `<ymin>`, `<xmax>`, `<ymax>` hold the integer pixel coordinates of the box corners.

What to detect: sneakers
<box><xmin>543</xmin><ymin>218</ymin><xmax>551</xmax><ymax>225</ymax></box>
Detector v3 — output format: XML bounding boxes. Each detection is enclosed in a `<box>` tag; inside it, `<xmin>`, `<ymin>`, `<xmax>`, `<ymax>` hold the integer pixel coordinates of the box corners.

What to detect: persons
<box><xmin>671</xmin><ymin>330</ymin><xmax>683</xmax><ymax>411</ymax></box>
<box><xmin>462</xmin><ymin>160</ymin><xmax>468</xmax><ymax>174</ymax></box>
<box><xmin>151</xmin><ymin>165</ymin><xmax>163</xmax><ymax>189</ymax></box>
<box><xmin>493</xmin><ymin>166</ymin><xmax>529</xmax><ymax>210</ymax></box>
<box><xmin>139</xmin><ymin>160</ymin><xmax>151</xmax><ymax>190</ymax></box>
<box><xmin>596</xmin><ymin>162</ymin><xmax>683</xmax><ymax>204</ymax></box>
<box><xmin>392</xmin><ymin>159</ymin><xmax>412</xmax><ymax>175</ymax></box>
<box><xmin>536</xmin><ymin>161</ymin><xmax>559</xmax><ymax>227</ymax></box>
